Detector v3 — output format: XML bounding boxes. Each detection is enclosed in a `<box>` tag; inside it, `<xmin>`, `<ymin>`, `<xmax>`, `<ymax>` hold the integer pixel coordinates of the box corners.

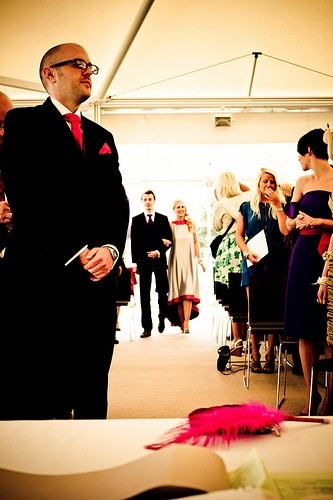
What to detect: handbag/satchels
<box><xmin>210</xmin><ymin>235</ymin><xmax>223</xmax><ymax>259</ymax></box>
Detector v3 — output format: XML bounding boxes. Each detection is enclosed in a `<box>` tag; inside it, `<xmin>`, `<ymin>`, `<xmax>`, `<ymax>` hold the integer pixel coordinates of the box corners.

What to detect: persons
<box><xmin>316</xmin><ymin>123</ymin><xmax>333</xmax><ymax>346</ymax></box>
<box><xmin>285</xmin><ymin>128</ymin><xmax>333</xmax><ymax>416</ymax></box>
<box><xmin>0</xmin><ymin>43</ymin><xmax>137</xmax><ymax>420</ymax></box>
<box><xmin>226</xmin><ymin>164</ymin><xmax>294</xmax><ymax>342</ymax></box>
<box><xmin>212</xmin><ymin>172</ymin><xmax>248</xmax><ymax>357</ymax></box>
<box><xmin>235</xmin><ymin>168</ymin><xmax>292</xmax><ymax>374</ymax></box>
<box><xmin>130</xmin><ymin>190</ymin><xmax>174</xmax><ymax>338</ymax></box>
<box><xmin>162</xmin><ymin>199</ymin><xmax>205</xmax><ymax>334</ymax></box>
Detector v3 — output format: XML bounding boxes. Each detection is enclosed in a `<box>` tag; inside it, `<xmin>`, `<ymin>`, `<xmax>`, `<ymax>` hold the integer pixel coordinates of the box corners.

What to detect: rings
<box><xmin>317</xmin><ymin>299</ymin><xmax>318</xmax><ymax>303</ymax></box>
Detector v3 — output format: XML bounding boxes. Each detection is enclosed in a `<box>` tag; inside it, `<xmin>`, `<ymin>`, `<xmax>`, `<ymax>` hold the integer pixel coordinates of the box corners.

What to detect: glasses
<box><xmin>49</xmin><ymin>58</ymin><xmax>99</xmax><ymax>75</ymax></box>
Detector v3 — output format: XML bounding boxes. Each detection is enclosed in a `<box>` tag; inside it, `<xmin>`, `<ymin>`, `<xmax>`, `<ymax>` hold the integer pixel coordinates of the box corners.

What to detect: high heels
<box><xmin>250</xmin><ymin>353</ymin><xmax>262</xmax><ymax>373</ymax></box>
<box><xmin>242</xmin><ymin>339</ymin><xmax>261</xmax><ymax>354</ymax></box>
<box><xmin>184</xmin><ymin>322</ymin><xmax>189</xmax><ymax>333</ymax></box>
<box><xmin>298</xmin><ymin>395</ymin><xmax>322</xmax><ymax>416</ymax></box>
<box><xmin>231</xmin><ymin>338</ymin><xmax>243</xmax><ymax>357</ymax></box>
<box><xmin>263</xmin><ymin>353</ymin><xmax>276</xmax><ymax>374</ymax></box>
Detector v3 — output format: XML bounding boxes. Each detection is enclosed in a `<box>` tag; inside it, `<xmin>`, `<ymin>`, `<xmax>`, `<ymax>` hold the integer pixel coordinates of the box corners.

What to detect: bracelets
<box><xmin>198</xmin><ymin>258</ymin><xmax>202</xmax><ymax>264</ymax></box>
<box><xmin>276</xmin><ymin>208</ymin><xmax>283</xmax><ymax>212</ymax></box>
<box><xmin>312</xmin><ymin>277</ymin><xmax>327</xmax><ymax>286</ymax></box>
<box><xmin>242</xmin><ymin>251</ymin><xmax>249</xmax><ymax>259</ymax></box>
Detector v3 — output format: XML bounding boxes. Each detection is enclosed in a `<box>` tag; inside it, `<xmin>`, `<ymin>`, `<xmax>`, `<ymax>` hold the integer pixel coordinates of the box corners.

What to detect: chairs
<box><xmin>0</xmin><ymin>443</ymin><xmax>229</xmax><ymax>500</ymax></box>
<box><xmin>208</xmin><ymin>267</ymin><xmax>332</xmax><ymax>415</ymax></box>
<box><xmin>117</xmin><ymin>276</ymin><xmax>135</xmax><ymax>340</ymax></box>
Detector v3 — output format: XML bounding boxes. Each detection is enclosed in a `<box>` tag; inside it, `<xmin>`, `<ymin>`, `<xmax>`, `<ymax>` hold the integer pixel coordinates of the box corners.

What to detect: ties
<box><xmin>63</xmin><ymin>113</ymin><xmax>83</xmax><ymax>151</ymax></box>
<box><xmin>147</xmin><ymin>214</ymin><xmax>152</xmax><ymax>224</ymax></box>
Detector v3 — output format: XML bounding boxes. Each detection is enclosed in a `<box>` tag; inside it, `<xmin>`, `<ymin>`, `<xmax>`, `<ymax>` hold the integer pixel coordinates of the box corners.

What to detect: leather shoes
<box><xmin>141</xmin><ymin>330</ymin><xmax>151</xmax><ymax>338</ymax></box>
<box><xmin>158</xmin><ymin>319</ymin><xmax>165</xmax><ymax>333</ymax></box>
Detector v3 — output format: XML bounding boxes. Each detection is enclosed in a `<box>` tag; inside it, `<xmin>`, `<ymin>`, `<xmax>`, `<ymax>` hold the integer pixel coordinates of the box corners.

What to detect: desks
<box><xmin>1</xmin><ymin>416</ymin><xmax>333</xmax><ymax>499</ymax></box>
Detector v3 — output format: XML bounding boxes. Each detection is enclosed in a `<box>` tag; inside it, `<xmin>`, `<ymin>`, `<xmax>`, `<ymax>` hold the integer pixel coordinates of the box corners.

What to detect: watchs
<box><xmin>107</xmin><ymin>246</ymin><xmax>118</xmax><ymax>260</ymax></box>
